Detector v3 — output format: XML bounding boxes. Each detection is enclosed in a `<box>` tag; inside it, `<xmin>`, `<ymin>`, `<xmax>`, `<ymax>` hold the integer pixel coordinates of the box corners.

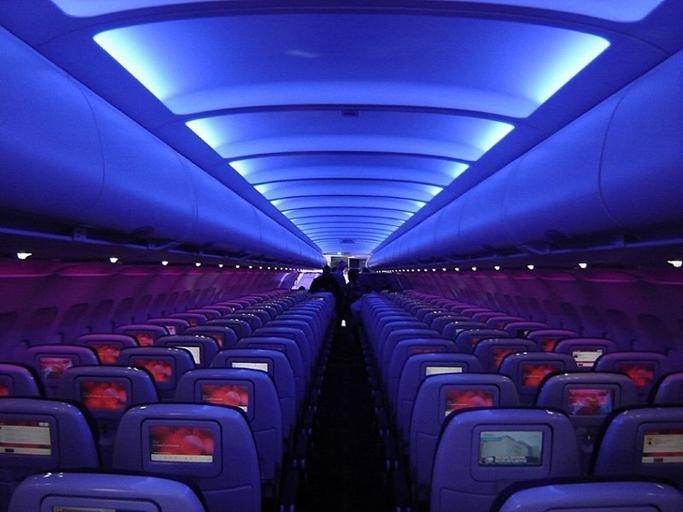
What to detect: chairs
<box><xmin>359</xmin><ymin>286</ymin><xmax>682</xmax><ymax>512</ymax></box>
<box><xmin>0</xmin><ymin>288</ymin><xmax>336</xmax><ymax>512</ymax></box>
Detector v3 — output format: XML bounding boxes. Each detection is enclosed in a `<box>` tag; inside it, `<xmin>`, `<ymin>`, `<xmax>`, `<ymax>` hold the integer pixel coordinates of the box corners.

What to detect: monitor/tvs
<box><xmin>331</xmin><ymin>257</ymin><xmax>348</xmax><ymax>271</ymax></box>
<box><xmin>195</xmin><ymin>380</ymin><xmax>254</xmax><ymax>421</ymax></box>
<box><xmin>35</xmin><ymin>354</ymin><xmax>80</xmax><ymax>384</ymax></box>
<box><xmin>2</xmin><ymin>413</ymin><xmax>59</xmax><ymax>469</ymax></box>
<box><xmin>191</xmin><ymin>322</ymin><xmax>199</xmax><ymax>326</ymax></box>
<box><xmin>174</xmin><ymin>345</ymin><xmax>203</xmax><ymax>367</ymax></box>
<box><xmin>227</xmin><ymin>358</ymin><xmax>273</xmax><ymax>376</ymax></box>
<box><xmin>88</xmin><ymin>341</ymin><xmax>121</xmax><ymax>365</ymax></box>
<box><xmin>1</xmin><ymin>375</ymin><xmax>13</xmax><ymax>397</ymax></box>
<box><xmin>193</xmin><ymin>331</ymin><xmax>225</xmax><ymax>348</ymax></box>
<box><xmin>423</xmin><ymin>329</ymin><xmax>683</xmax><ymax>481</ymax></box>
<box><xmin>130</xmin><ymin>357</ymin><xmax>175</xmax><ymax>390</ymax></box>
<box><xmin>40</xmin><ymin>496</ymin><xmax>158</xmax><ymax>511</ymax></box>
<box><xmin>126</xmin><ymin>331</ymin><xmax>154</xmax><ymax>346</ymax></box>
<box><xmin>142</xmin><ymin>419</ymin><xmax>222</xmax><ymax>477</ymax></box>
<box><xmin>75</xmin><ymin>377</ymin><xmax>133</xmax><ymax>418</ymax></box>
<box><xmin>164</xmin><ymin>325</ymin><xmax>177</xmax><ymax>336</ymax></box>
<box><xmin>349</xmin><ymin>258</ymin><xmax>360</xmax><ymax>270</ymax></box>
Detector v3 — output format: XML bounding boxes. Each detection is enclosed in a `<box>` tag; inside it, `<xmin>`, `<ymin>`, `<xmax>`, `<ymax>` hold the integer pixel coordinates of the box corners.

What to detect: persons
<box><xmin>308</xmin><ymin>260</ymin><xmax>369</xmax><ymax>320</ymax></box>
